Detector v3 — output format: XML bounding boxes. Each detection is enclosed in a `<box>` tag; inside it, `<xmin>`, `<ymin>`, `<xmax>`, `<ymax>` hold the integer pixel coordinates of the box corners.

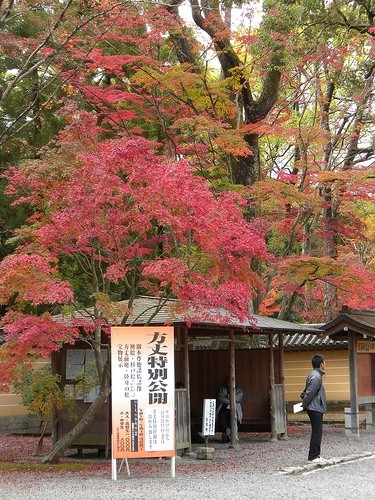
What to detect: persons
<box><xmin>215</xmin><ymin>378</ymin><xmax>244</xmax><ymax>442</ymax></box>
<box><xmin>300</xmin><ymin>353</ymin><xmax>328</xmax><ymax>464</ymax></box>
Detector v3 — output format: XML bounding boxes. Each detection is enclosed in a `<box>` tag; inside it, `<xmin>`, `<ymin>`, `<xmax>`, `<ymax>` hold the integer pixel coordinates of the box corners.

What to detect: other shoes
<box><xmin>225</xmin><ymin>428</ymin><xmax>232</xmax><ymax>438</ymax></box>
<box><xmin>308</xmin><ymin>457</ymin><xmax>330</xmax><ymax>465</ymax></box>
